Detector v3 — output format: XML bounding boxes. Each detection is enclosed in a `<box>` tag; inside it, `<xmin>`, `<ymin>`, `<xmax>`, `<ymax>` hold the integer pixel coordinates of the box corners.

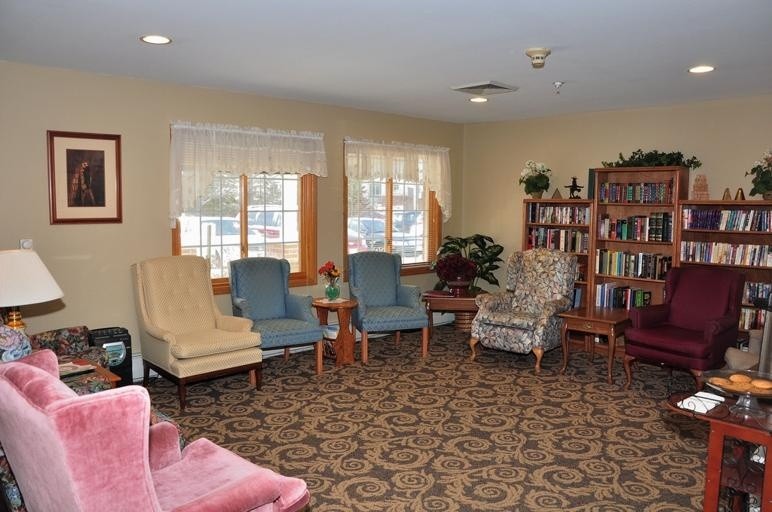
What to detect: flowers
<box><xmin>319</xmin><ymin>260</ymin><xmax>345</xmax><ymax>284</ymax></box>
<box><xmin>519</xmin><ymin>159</ymin><xmax>552</xmax><ymax>195</ymax></box>
<box><xmin>744</xmin><ymin>150</ymin><xmax>772</xmax><ymax>196</ymax></box>
<box><xmin>435</xmin><ymin>255</ymin><xmax>477</xmax><ymax>281</ymax></box>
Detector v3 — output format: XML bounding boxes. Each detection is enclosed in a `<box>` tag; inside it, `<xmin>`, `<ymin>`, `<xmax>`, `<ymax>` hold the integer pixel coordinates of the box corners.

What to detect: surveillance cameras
<box><xmin>525</xmin><ymin>48</ymin><xmax>551</xmax><ymax>69</ymax></box>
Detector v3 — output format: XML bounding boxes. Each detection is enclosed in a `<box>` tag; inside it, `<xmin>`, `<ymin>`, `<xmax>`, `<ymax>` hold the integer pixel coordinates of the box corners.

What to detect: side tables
<box><xmin>421</xmin><ymin>291</ymin><xmax>489</xmax><ymax>338</ymax></box>
<box><xmin>664</xmin><ymin>388</ymin><xmax>772</xmax><ymax>512</ymax></box>
<box><xmin>59</xmin><ymin>359</ymin><xmax>121</xmax><ymax>389</ymax></box>
<box><xmin>312</xmin><ymin>297</ymin><xmax>358</xmax><ymax>368</ymax></box>
<box><xmin>555</xmin><ymin>307</ymin><xmax>631</xmax><ymax>385</ymax></box>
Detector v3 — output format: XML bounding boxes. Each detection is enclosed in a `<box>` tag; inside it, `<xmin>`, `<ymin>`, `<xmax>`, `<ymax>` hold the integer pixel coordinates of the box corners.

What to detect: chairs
<box><xmin>348</xmin><ymin>251</ymin><xmax>429</xmax><ymax>363</ymax></box>
<box><xmin>624</xmin><ymin>266</ymin><xmax>746</xmax><ymax>392</ymax></box>
<box><xmin>130</xmin><ymin>255</ymin><xmax>262</xmax><ymax>413</ymax></box>
<box><xmin>227</xmin><ymin>257</ymin><xmax>323</xmax><ymax>386</ymax></box>
<box><xmin>470</xmin><ymin>248</ymin><xmax>577</xmax><ymax>373</ymax></box>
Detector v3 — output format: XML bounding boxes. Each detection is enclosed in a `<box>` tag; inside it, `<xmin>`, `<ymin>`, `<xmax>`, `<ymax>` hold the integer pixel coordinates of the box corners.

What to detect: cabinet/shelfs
<box><xmin>675</xmin><ymin>200</ymin><xmax>772</xmax><ymax>346</ymax></box>
<box><xmin>589</xmin><ymin>166</ymin><xmax>689</xmax><ymax>358</ymax></box>
<box><xmin>521</xmin><ymin>199</ymin><xmax>593</xmax><ymax>352</ymax></box>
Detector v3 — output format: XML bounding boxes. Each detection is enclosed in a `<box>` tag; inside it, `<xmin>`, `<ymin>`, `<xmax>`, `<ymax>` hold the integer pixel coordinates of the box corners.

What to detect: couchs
<box><xmin>0</xmin><ymin>316</ymin><xmax>109</xmax><ymax>395</ymax></box>
<box><xmin>0</xmin><ymin>349</ymin><xmax>310</xmax><ymax>511</ymax></box>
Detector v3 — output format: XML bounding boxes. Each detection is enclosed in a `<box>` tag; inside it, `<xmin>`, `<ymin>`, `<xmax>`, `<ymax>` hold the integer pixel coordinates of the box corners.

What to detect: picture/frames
<box><xmin>47</xmin><ymin>130</ymin><xmax>123</xmax><ymax>225</ymax></box>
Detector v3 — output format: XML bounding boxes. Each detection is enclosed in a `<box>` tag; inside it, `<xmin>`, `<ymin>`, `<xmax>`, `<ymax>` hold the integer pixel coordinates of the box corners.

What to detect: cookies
<box><xmin>709</xmin><ymin>373</ymin><xmax>772</xmax><ymax>395</ymax></box>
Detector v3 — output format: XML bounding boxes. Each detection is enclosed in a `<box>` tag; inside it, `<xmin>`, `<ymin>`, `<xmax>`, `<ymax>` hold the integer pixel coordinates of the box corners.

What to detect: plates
<box><xmin>702</xmin><ymin>370</ymin><xmax>772</xmax><ymax>418</ymax></box>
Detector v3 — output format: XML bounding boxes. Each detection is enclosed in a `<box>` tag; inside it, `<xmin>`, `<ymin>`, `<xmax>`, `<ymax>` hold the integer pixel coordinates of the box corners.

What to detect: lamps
<box><xmin>0</xmin><ymin>249</ymin><xmax>65</xmax><ymax>332</ymax></box>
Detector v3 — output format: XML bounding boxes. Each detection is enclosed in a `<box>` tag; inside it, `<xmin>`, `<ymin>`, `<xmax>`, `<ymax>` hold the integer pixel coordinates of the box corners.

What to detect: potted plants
<box><xmin>430</xmin><ymin>234</ymin><xmax>505</xmax><ymax>332</ymax></box>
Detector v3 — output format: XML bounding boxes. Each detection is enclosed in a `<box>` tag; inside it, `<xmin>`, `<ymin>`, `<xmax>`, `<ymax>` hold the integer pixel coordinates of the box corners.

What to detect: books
<box><xmin>576</xmin><ymin>263</ymin><xmax>586</xmax><ymax>282</ymax></box>
<box><xmin>597</xmin><ymin>282</ymin><xmax>651</xmax><ymax>312</ymax></box>
<box><xmin>527</xmin><ymin>227</ymin><xmax>588</xmax><ymax>253</ymax></box>
<box><xmin>58</xmin><ymin>358</ymin><xmax>97</xmax><ymax>376</ymax></box>
<box><xmin>682</xmin><ymin>209</ymin><xmax>772</xmax><ymax>232</ymax></box>
<box><xmin>739</xmin><ymin>308</ymin><xmax>767</xmax><ymax>331</ymax></box>
<box><xmin>742</xmin><ymin>281</ymin><xmax>772</xmax><ymax>305</ymax></box>
<box><xmin>596</xmin><ymin>248</ymin><xmax>673</xmax><ymax>280</ymax></box>
<box><xmin>597</xmin><ymin>211</ymin><xmax>673</xmax><ymax>242</ymax></box>
<box><xmin>528</xmin><ymin>202</ymin><xmax>590</xmax><ymax>225</ymax></box>
<box><xmin>737</xmin><ymin>339</ymin><xmax>749</xmax><ymax>353</ymax></box>
<box><xmin>600</xmin><ymin>175</ymin><xmax>674</xmax><ymax>204</ymax></box>
<box><xmin>680</xmin><ymin>240</ymin><xmax>772</xmax><ymax>266</ymax></box>
<box><xmin>571</xmin><ymin>288</ymin><xmax>582</xmax><ymax>310</ymax></box>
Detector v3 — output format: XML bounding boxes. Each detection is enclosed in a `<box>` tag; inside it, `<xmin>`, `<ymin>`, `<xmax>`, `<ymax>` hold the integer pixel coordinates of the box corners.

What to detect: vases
<box><xmin>762</xmin><ymin>191</ymin><xmax>772</xmax><ymax>200</ymax></box>
<box><xmin>530</xmin><ymin>191</ymin><xmax>543</xmax><ymax>199</ymax></box>
<box><xmin>324</xmin><ymin>284</ymin><xmax>340</xmax><ymax>301</ymax></box>
<box><xmin>447</xmin><ymin>281</ymin><xmax>470</xmax><ymax>296</ymax></box>
